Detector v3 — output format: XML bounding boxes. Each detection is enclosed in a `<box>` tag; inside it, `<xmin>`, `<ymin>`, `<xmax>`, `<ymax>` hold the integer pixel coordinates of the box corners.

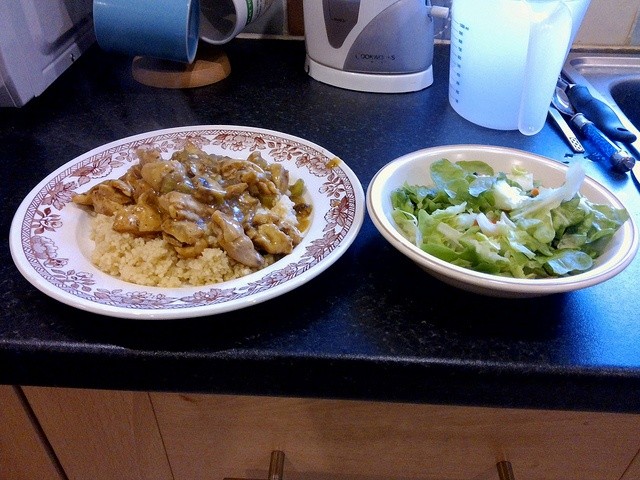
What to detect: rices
<box><xmin>88</xmin><ymin>187</ymin><xmax>298</xmax><ymax>288</ymax></box>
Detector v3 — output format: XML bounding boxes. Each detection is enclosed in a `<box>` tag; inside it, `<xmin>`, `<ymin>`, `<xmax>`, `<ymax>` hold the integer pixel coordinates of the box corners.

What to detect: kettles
<box><xmin>301</xmin><ymin>0</ymin><xmax>450</xmax><ymax>93</ymax></box>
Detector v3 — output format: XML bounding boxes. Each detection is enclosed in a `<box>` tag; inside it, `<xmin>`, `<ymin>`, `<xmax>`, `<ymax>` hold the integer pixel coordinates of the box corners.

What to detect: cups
<box><xmin>92</xmin><ymin>0</ymin><xmax>202</xmax><ymax>66</ymax></box>
<box><xmin>199</xmin><ymin>0</ymin><xmax>273</xmax><ymax>45</ymax></box>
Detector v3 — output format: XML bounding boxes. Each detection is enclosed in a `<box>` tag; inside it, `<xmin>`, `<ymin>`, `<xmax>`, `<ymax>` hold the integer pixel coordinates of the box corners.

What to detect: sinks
<box><xmin>563</xmin><ymin>51</ymin><xmax>640</xmax><ymax>188</ymax></box>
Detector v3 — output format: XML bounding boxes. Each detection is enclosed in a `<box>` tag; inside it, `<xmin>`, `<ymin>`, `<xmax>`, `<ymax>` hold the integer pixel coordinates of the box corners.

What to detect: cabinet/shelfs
<box><xmin>0</xmin><ymin>384</ymin><xmax>176</xmax><ymax>480</ymax></box>
<box><xmin>150</xmin><ymin>393</ymin><xmax>639</xmax><ymax>480</ymax></box>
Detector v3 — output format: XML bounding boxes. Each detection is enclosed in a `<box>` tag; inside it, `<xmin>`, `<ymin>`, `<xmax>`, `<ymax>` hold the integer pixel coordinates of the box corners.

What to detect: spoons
<box><xmin>552</xmin><ymin>86</ymin><xmax>635</xmax><ymax>173</ymax></box>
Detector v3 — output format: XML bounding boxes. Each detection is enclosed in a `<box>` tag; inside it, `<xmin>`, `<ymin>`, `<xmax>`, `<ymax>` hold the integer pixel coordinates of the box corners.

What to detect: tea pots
<box><xmin>448</xmin><ymin>0</ymin><xmax>593</xmax><ymax>136</ymax></box>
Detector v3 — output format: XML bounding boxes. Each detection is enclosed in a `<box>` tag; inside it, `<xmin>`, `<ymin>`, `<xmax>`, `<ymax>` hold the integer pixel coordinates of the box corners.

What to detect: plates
<box><xmin>8</xmin><ymin>125</ymin><xmax>366</xmax><ymax>319</ymax></box>
<box><xmin>366</xmin><ymin>145</ymin><xmax>638</xmax><ymax>298</ymax></box>
